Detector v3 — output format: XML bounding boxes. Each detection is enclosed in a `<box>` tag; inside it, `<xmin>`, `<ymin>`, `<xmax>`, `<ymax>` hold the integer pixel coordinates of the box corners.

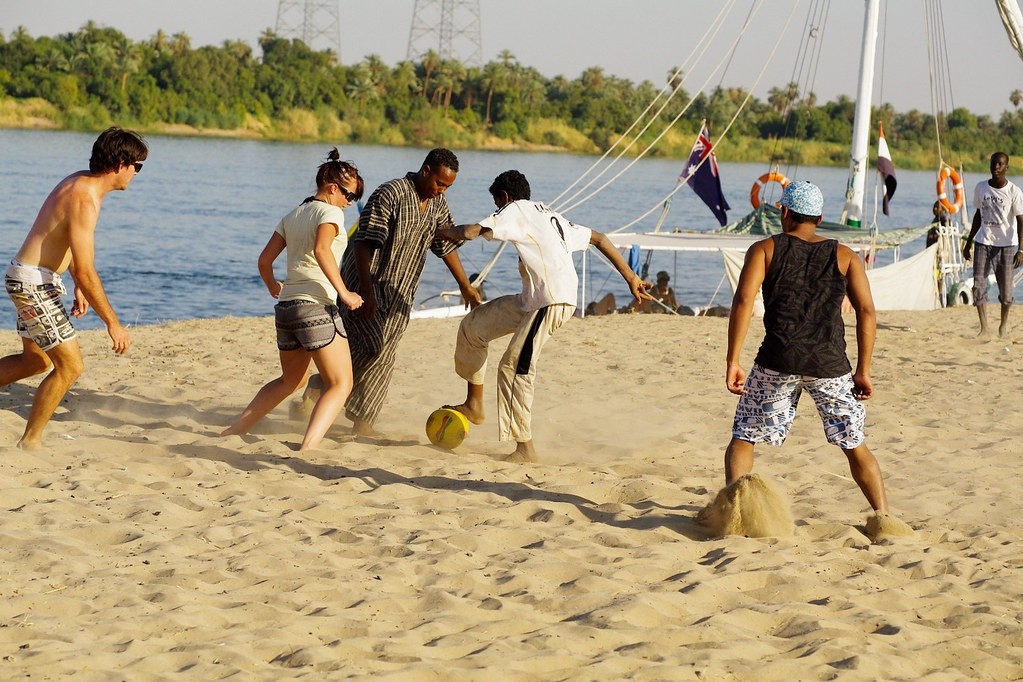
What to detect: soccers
<box><xmin>426</xmin><ymin>406</ymin><xmax>467</xmax><ymax>449</ymax></box>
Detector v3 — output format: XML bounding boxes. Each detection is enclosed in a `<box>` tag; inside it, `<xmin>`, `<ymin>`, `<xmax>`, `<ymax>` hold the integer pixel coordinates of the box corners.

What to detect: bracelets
<box><xmin>1018</xmin><ymin>249</ymin><xmax>1023</xmax><ymax>253</ymax></box>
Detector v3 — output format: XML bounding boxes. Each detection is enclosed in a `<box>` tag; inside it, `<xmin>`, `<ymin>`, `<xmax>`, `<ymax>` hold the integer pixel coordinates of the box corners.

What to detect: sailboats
<box><xmin>409</xmin><ymin>2</ymin><xmax>1023</xmax><ymax>322</ymax></box>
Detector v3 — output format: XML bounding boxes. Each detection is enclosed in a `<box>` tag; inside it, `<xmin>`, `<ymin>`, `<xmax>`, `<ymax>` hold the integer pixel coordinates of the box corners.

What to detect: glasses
<box><xmin>131</xmin><ymin>162</ymin><xmax>143</xmax><ymax>172</ymax></box>
<box><xmin>335</xmin><ymin>182</ymin><xmax>355</xmax><ymax>203</ymax></box>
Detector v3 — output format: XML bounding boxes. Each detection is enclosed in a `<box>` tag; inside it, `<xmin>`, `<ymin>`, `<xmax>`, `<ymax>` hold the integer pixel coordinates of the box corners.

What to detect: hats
<box><xmin>656</xmin><ymin>270</ymin><xmax>671</xmax><ymax>282</ymax></box>
<box><xmin>775</xmin><ymin>181</ymin><xmax>823</xmax><ymax>216</ymax></box>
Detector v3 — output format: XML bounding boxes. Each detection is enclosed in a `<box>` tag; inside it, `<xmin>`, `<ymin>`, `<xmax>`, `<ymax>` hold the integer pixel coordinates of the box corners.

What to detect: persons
<box><xmin>221</xmin><ymin>145</ymin><xmax>367</xmax><ymax>452</ymax></box>
<box><xmin>0</xmin><ymin>126</ymin><xmax>149</xmax><ymax>452</ymax></box>
<box><xmin>962</xmin><ymin>152</ymin><xmax>1023</xmax><ymax>335</ymax></box>
<box><xmin>299</xmin><ymin>146</ymin><xmax>483</xmax><ymax>438</ymax></box>
<box><xmin>434</xmin><ymin>169</ymin><xmax>653</xmax><ymax>461</ymax></box>
<box><xmin>692</xmin><ymin>175</ymin><xmax>891</xmax><ymax>533</ymax></box>
<box><xmin>651</xmin><ymin>270</ymin><xmax>679</xmax><ymax>314</ymax></box>
<box><xmin>460</xmin><ymin>273</ymin><xmax>488</xmax><ymax>305</ymax></box>
<box><xmin>924</xmin><ymin>198</ymin><xmax>953</xmax><ymax>307</ymax></box>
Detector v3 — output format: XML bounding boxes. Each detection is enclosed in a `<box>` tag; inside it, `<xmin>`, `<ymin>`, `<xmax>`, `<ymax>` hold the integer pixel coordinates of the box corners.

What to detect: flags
<box><xmin>873</xmin><ymin>120</ymin><xmax>900</xmax><ymax>215</ymax></box>
<box><xmin>678</xmin><ymin>122</ymin><xmax>731</xmax><ymax>228</ymax></box>
